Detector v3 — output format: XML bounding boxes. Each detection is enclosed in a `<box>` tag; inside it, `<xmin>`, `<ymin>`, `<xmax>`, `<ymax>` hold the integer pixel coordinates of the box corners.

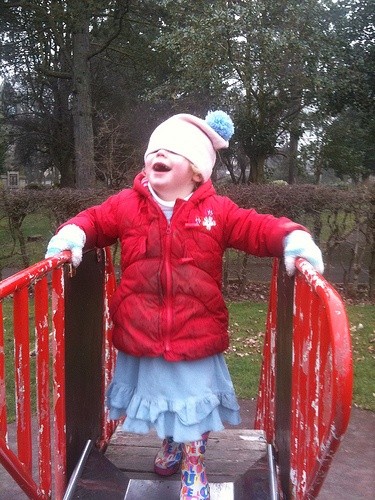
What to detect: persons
<box><xmin>41</xmin><ymin>110</ymin><xmax>324</xmax><ymax>500</ymax></box>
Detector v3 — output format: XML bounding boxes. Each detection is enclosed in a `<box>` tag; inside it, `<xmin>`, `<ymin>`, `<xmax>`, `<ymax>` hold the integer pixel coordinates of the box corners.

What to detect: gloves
<box><xmin>44</xmin><ymin>224</ymin><xmax>86</xmax><ymax>268</ymax></box>
<box><xmin>283</xmin><ymin>229</ymin><xmax>325</xmax><ymax>276</ymax></box>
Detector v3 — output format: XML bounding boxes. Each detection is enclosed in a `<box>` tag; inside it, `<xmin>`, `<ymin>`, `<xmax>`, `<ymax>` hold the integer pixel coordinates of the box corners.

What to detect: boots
<box><xmin>154</xmin><ymin>436</ymin><xmax>183</xmax><ymax>476</ymax></box>
<box><xmin>179</xmin><ymin>432</ymin><xmax>211</xmax><ymax>500</ymax></box>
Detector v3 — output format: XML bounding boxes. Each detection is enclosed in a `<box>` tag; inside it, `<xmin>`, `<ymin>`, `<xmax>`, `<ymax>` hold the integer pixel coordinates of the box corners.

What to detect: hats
<box><xmin>144</xmin><ymin>109</ymin><xmax>235</xmax><ymax>183</ymax></box>
